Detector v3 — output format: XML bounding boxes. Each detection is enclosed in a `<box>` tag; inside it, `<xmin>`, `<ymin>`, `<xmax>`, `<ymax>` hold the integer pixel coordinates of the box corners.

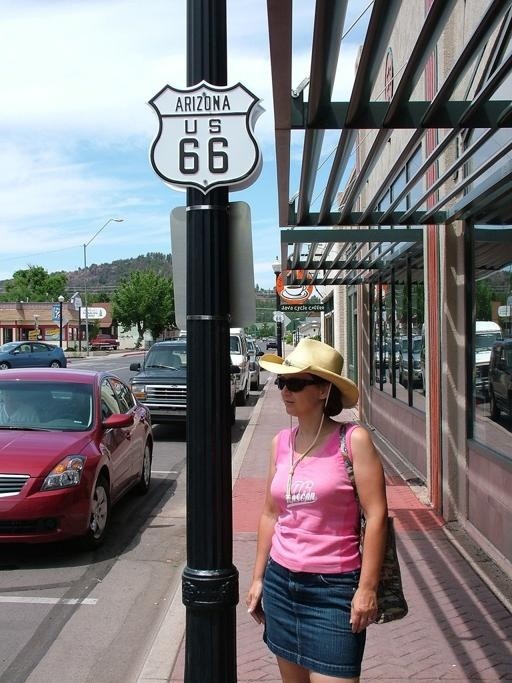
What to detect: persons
<box><xmin>246</xmin><ymin>337</ymin><xmax>388</xmax><ymax>683</ymax></box>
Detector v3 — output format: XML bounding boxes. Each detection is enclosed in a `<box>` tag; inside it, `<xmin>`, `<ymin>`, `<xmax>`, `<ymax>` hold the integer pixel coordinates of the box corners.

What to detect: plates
<box><xmin>281</xmin><ymin>289</ymin><xmax>309</xmax><ymax>300</ymax></box>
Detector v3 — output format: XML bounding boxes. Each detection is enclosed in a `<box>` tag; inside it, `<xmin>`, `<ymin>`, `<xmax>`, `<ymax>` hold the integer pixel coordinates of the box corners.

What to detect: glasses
<box><xmin>274</xmin><ymin>373</ymin><xmax>329</xmax><ymax>393</ymax></box>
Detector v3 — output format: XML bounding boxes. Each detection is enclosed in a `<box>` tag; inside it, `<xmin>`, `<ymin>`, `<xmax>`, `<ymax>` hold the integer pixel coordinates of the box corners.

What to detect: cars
<box><xmin>88</xmin><ymin>332</ymin><xmax>120</xmax><ymax>349</ymax></box>
<box><xmin>0</xmin><ymin>340</ymin><xmax>66</xmax><ymax>370</ymax></box>
<box><xmin>0</xmin><ymin>368</ymin><xmax>154</xmax><ymax>550</ymax></box>
<box><xmin>373</xmin><ymin>317</ymin><xmax>512</xmax><ymax>431</ymax></box>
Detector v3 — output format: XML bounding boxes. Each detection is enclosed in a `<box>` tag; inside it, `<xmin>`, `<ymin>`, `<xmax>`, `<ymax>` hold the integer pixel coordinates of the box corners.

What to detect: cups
<box><xmin>284</xmin><ymin>284</ymin><xmax>306</xmax><ymax>295</ymax></box>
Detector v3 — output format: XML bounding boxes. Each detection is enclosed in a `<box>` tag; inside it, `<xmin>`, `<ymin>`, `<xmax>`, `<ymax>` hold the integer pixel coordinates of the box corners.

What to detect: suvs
<box><xmin>123</xmin><ymin>326</ymin><xmax>279</xmax><ymax>428</ymax></box>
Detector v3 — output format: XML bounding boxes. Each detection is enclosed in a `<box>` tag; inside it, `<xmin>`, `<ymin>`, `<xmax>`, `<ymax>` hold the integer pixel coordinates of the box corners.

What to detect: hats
<box><xmin>258</xmin><ymin>337</ymin><xmax>361</xmax><ymax>409</ymax></box>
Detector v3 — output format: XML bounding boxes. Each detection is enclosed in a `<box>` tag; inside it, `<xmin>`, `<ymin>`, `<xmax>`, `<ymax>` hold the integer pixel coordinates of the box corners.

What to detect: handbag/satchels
<box><xmin>339</xmin><ymin>420</ymin><xmax>409</xmax><ymax>625</ymax></box>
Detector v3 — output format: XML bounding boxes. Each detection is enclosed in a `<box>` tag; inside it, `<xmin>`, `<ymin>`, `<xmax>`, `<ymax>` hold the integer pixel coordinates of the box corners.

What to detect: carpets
<box><xmin>230</xmin><ymin>475</ymin><xmax>446</xmax><ymax>532</ymax></box>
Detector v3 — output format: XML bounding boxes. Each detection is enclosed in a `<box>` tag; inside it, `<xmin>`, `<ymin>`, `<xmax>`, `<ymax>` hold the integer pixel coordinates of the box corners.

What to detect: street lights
<box><xmin>83</xmin><ymin>215</ymin><xmax>126</xmax><ymax>358</ymax></box>
<box><xmin>57</xmin><ymin>294</ymin><xmax>65</xmax><ymax>350</ymax></box>
<box><xmin>271</xmin><ymin>255</ymin><xmax>282</xmax><ymax>356</ymax></box>
